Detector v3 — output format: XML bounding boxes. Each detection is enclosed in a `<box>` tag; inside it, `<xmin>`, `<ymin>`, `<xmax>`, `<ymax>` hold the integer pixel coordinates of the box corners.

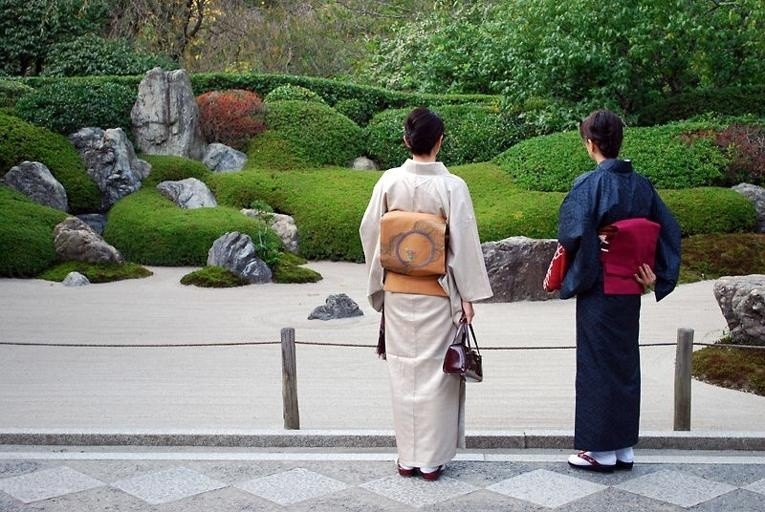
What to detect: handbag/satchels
<box><xmin>443</xmin><ymin>343</ymin><xmax>481</xmax><ymax>382</ymax></box>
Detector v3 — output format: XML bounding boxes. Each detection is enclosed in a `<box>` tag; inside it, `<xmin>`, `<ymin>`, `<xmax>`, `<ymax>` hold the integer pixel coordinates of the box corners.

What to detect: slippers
<box><xmin>398</xmin><ymin>460</ymin><xmax>414</xmax><ymax>476</ymax></box>
<box><xmin>423</xmin><ymin>465</ymin><xmax>444</xmax><ymax>480</ymax></box>
<box><xmin>568</xmin><ymin>451</ymin><xmax>633</xmax><ymax>472</ymax></box>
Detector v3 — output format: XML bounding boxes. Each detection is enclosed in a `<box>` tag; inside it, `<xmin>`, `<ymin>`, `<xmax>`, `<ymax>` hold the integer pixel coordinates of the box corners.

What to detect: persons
<box><xmin>358</xmin><ymin>104</ymin><xmax>494</xmax><ymax>482</ymax></box>
<box><xmin>540</xmin><ymin>109</ymin><xmax>682</xmax><ymax>473</ymax></box>
